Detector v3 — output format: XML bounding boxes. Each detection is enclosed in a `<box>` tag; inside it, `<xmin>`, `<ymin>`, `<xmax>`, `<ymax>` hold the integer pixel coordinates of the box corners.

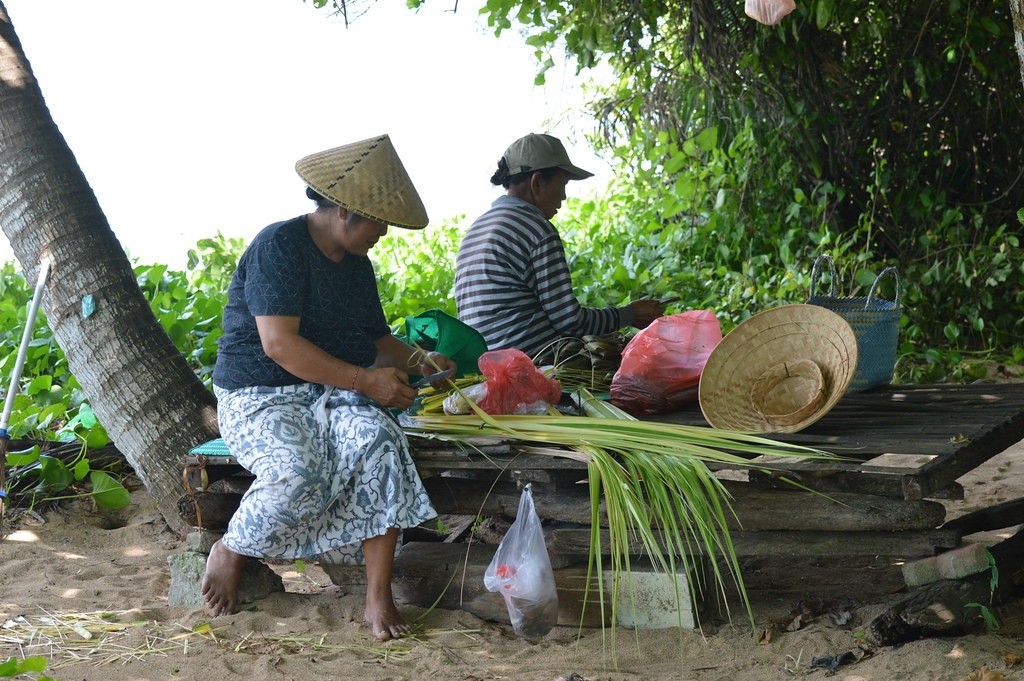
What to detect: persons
<box><xmin>200</xmin><ymin>132</ymin><xmax>455</xmax><ymax>642</ymax></box>
<box><xmin>455</xmin><ymin>132</ymin><xmax>664</xmax><ymax>368</ymax></box>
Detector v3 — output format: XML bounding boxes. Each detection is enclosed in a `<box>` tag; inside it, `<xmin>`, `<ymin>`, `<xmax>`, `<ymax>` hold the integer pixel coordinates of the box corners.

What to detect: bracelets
<box><xmin>351</xmin><ymin>366</ymin><xmax>360</xmax><ymax>390</ymax></box>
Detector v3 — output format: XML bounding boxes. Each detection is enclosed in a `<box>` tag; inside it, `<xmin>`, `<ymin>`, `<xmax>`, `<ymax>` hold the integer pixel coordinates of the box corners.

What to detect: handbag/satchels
<box><xmin>387</xmin><ymin>308</ymin><xmax>489</xmax><ymax>379</ymax></box>
<box><xmin>609</xmin><ymin>309</ymin><xmax>722</xmax><ymax>409</ymax></box>
<box><xmin>477</xmin><ymin>348</ymin><xmax>562</xmax><ymax>413</ymax></box>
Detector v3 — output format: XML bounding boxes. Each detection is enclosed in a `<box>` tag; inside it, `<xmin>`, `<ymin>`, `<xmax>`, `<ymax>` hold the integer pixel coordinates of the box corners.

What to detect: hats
<box><xmin>698</xmin><ymin>303</ymin><xmax>858</xmax><ymax>434</ymax></box>
<box><xmin>502</xmin><ymin>133</ymin><xmax>595</xmax><ymax>181</ymax></box>
<box><xmin>804</xmin><ymin>254</ymin><xmax>905</xmax><ymax>393</ymax></box>
<box><xmin>295</xmin><ymin>134</ymin><xmax>430</xmax><ymax>230</ymax></box>
